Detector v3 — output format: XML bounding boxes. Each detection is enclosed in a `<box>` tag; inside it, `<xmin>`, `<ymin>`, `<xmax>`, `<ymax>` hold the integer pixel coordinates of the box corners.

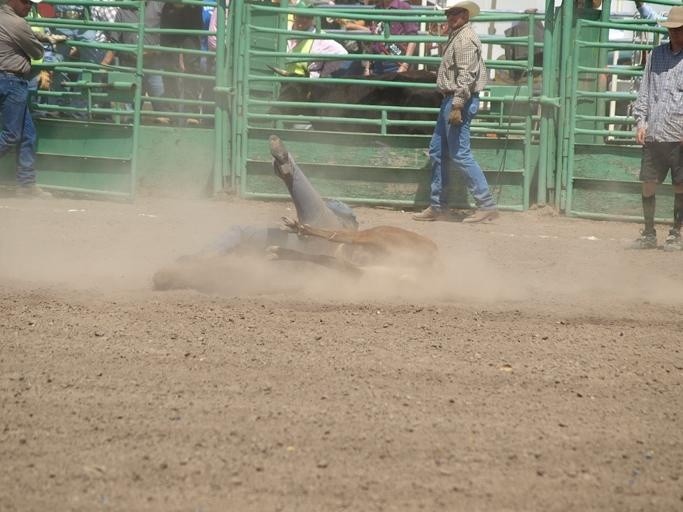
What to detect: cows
<box><xmin>143</xmin><ymin>212</ymin><xmax>441</xmax><ymax>294</ymax></box>
<box><xmin>258</xmin><ymin>64</ymin><xmax>443</xmax><ymax>134</ymax></box>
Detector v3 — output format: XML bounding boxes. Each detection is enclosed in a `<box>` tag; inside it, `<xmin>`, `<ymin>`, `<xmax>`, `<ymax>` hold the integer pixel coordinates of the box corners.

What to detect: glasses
<box><xmin>20</xmin><ymin>0</ymin><xmax>32</xmax><ymax>5</ymax></box>
<box><xmin>445</xmin><ymin>9</ymin><xmax>464</xmax><ymax>16</ymax></box>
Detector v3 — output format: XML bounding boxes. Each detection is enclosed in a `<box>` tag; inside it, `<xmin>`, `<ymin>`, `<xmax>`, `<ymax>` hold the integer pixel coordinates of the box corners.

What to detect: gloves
<box><xmin>447</xmin><ymin>104</ymin><xmax>464</xmax><ymax>127</ymax></box>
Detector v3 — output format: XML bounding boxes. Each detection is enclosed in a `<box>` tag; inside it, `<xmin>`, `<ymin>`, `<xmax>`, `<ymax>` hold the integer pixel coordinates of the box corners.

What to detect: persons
<box><xmin>632</xmin><ymin>6</ymin><xmax>683</xmax><ymax>252</ymax></box>
<box><xmin>411</xmin><ymin>0</ymin><xmax>500</xmax><ymax>225</ymax></box>
<box><xmin>25</xmin><ymin>1</ymin><xmax>669</xmax><ymax>127</ymax></box>
<box><xmin>267</xmin><ymin>134</ymin><xmax>359</xmax><ymax>254</ymax></box>
<box><xmin>0</xmin><ymin>0</ymin><xmax>67</xmax><ymax>198</ymax></box>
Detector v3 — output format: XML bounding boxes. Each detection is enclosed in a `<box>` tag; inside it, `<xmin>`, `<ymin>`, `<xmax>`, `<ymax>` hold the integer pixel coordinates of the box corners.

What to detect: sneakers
<box><xmin>268</xmin><ymin>134</ymin><xmax>292</xmax><ymax>176</ymax></box>
<box><xmin>21</xmin><ymin>182</ymin><xmax>52</xmax><ymax>200</ymax></box>
<box><xmin>636</xmin><ymin>228</ymin><xmax>657</xmax><ymax>249</ymax></box>
<box><xmin>411</xmin><ymin>206</ymin><xmax>448</xmax><ymax>221</ymax></box>
<box><xmin>154</xmin><ymin>116</ymin><xmax>199</xmax><ymax>126</ymax></box>
<box><xmin>463</xmin><ymin>209</ymin><xmax>501</xmax><ymax>225</ymax></box>
<box><xmin>664</xmin><ymin>229</ymin><xmax>683</xmax><ymax>252</ymax></box>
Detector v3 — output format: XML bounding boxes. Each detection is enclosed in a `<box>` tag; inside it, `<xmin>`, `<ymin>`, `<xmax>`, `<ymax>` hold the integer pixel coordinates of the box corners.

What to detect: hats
<box><xmin>436</xmin><ymin>0</ymin><xmax>480</xmax><ymax>17</ymax></box>
<box><xmin>658</xmin><ymin>6</ymin><xmax>683</xmax><ymax>28</ymax></box>
